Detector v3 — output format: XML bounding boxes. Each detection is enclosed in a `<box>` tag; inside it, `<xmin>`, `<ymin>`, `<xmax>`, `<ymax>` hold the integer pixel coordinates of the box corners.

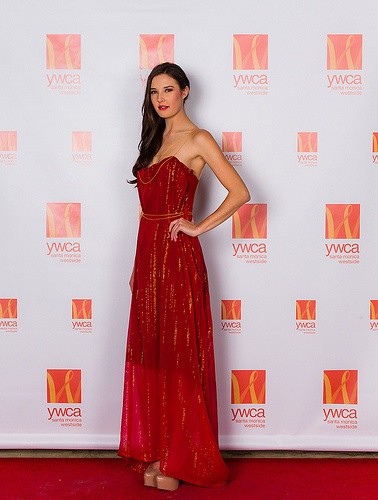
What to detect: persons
<box><xmin>117</xmin><ymin>62</ymin><xmax>251</xmax><ymax>491</ymax></box>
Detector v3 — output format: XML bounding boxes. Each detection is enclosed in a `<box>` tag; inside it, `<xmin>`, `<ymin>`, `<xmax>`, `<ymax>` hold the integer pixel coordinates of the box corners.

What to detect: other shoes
<box><xmin>144</xmin><ymin>462</ymin><xmax>160</xmax><ymax>487</ymax></box>
<box><xmin>156</xmin><ymin>471</ymin><xmax>179</xmax><ymax>490</ymax></box>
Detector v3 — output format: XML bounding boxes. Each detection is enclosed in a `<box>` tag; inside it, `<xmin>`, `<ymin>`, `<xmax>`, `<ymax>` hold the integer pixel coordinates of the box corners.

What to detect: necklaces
<box><xmin>138</xmin><ymin>131</ymin><xmax>195</xmax><ymax>184</ymax></box>
